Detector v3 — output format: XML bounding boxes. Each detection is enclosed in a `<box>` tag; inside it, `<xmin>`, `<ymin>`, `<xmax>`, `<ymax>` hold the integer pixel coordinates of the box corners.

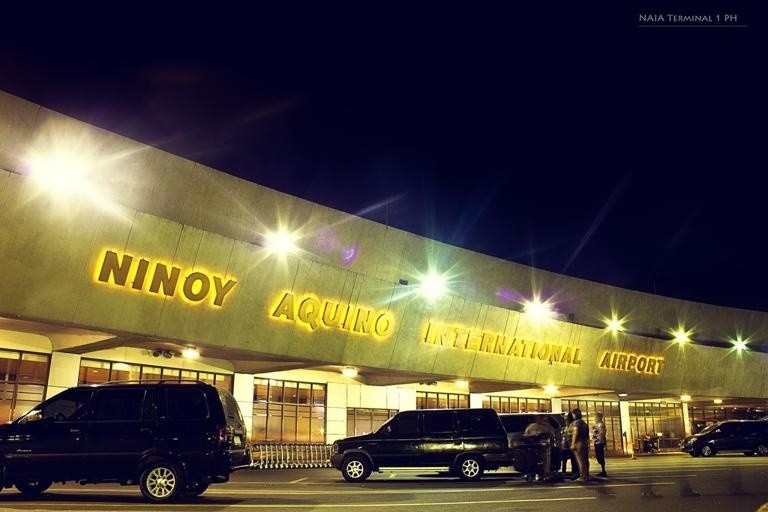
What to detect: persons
<box><xmin>562</xmin><ymin>408</ymin><xmax>608</xmax><ymax>483</ymax></box>
<box><xmin>677</xmin><ymin>438</ymin><xmax>685</xmax><ymax>448</ymax></box>
<box><xmin>642</xmin><ymin>431</ymin><xmax>660</xmax><ymax>454</ymax></box>
<box><xmin>712</xmin><ymin>420</ymin><xmax>721</xmax><ymax>454</ymax></box>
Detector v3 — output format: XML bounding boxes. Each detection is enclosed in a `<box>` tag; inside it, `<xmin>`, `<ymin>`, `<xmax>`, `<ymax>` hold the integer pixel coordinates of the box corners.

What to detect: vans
<box><xmin>680</xmin><ymin>418</ymin><xmax>767</xmax><ymax>457</ymax></box>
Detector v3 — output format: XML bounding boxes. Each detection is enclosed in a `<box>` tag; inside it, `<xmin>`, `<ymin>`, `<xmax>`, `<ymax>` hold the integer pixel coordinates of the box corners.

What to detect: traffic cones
<box><xmin>630</xmin><ymin>451</ymin><xmax>636</xmax><ymax>460</ymax></box>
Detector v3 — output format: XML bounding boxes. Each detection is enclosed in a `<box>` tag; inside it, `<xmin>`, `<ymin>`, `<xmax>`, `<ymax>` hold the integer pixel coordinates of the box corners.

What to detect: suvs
<box><xmin>330</xmin><ymin>407</ymin><xmax>507</xmax><ymax>484</ymax></box>
<box><xmin>496</xmin><ymin>413</ymin><xmax>577</xmax><ymax>475</ymax></box>
<box><xmin>0</xmin><ymin>379</ymin><xmax>252</xmax><ymax>505</ymax></box>
